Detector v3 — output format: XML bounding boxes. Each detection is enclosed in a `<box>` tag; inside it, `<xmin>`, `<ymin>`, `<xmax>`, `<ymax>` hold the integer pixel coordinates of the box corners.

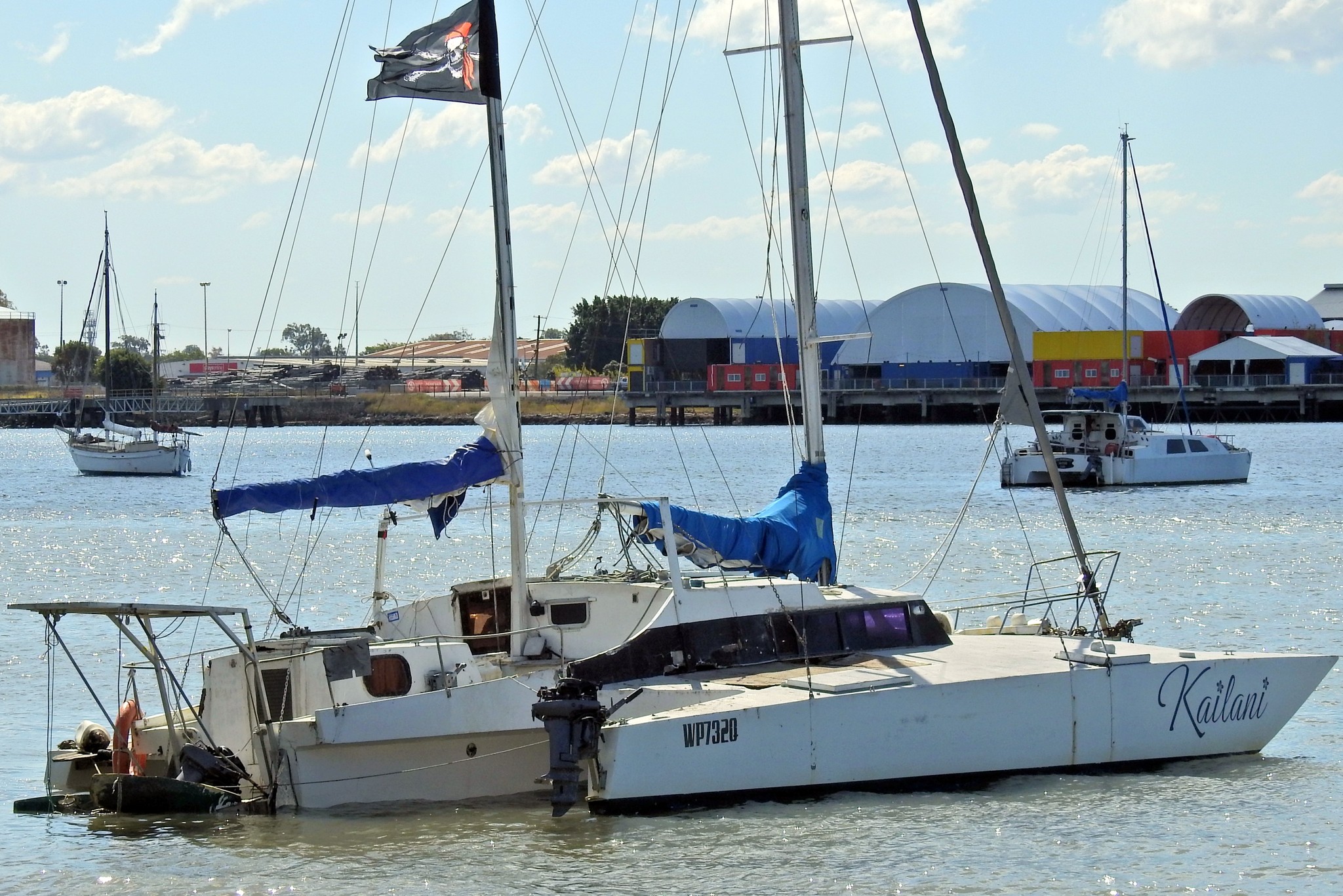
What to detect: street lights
<box><xmin>227</xmin><ymin>328</ymin><xmax>231</xmax><ymax>372</ymax></box>
<box><xmin>339</xmin><ymin>333</ymin><xmax>347</xmax><ymax>366</ymax></box>
<box><xmin>57</xmin><ymin>279</ymin><xmax>68</xmax><ymax>352</ymax></box>
<box><xmin>199</xmin><ymin>282</ymin><xmax>212</xmax><ymax>390</ymax></box>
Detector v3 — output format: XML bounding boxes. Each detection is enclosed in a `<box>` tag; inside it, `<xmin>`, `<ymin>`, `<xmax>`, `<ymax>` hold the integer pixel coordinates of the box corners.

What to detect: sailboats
<box><xmin>531</xmin><ymin>1</ymin><xmax>1343</xmax><ymax>817</ymax></box>
<box><xmin>5</xmin><ymin>0</ymin><xmax>858</xmax><ymax>832</ymax></box>
<box><xmin>52</xmin><ymin>210</ymin><xmax>204</xmax><ymax>476</ymax></box>
<box><xmin>999</xmin><ymin>122</ymin><xmax>1253</xmax><ymax>488</ymax></box>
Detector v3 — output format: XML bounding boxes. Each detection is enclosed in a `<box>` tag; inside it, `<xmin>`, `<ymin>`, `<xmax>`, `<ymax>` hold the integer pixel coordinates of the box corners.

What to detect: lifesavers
<box><xmin>112</xmin><ymin>699</ymin><xmax>147</xmax><ymax>776</ymax></box>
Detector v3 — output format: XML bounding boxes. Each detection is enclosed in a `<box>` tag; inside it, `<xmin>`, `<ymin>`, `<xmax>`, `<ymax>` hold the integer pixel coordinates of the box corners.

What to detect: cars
<box><xmin>618</xmin><ymin>377</ymin><xmax>628</xmax><ymax>391</ymax></box>
